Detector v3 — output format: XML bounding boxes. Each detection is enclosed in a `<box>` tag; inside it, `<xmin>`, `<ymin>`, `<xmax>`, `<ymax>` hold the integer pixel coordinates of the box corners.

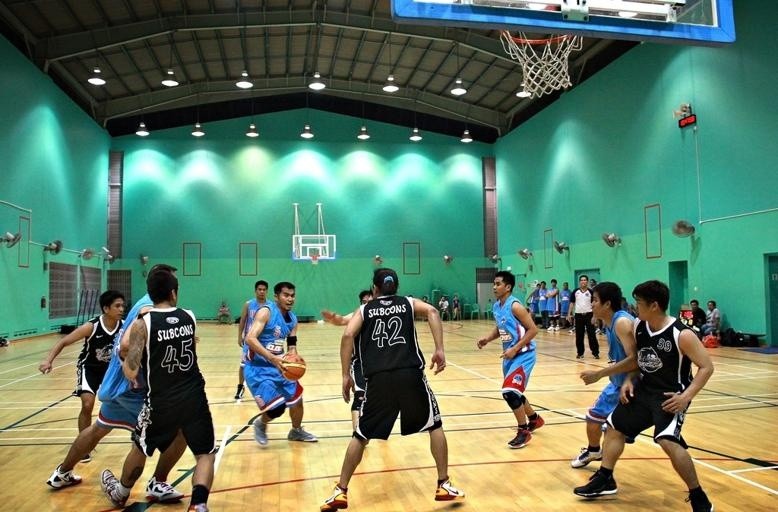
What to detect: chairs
<box><xmin>220</xmin><ymin>309</ymin><xmax>231</xmax><ymax>324</ymax></box>
<box><xmin>461</xmin><ymin>304</ymin><xmax>472</xmax><ymax>320</ymax></box>
<box><xmin>483</xmin><ymin>303</ymin><xmax>491</xmax><ymax>319</ymax></box>
<box><xmin>470</xmin><ymin>304</ymin><xmax>482</xmax><ymax>320</ymax></box>
<box><xmin>451</xmin><ymin>310</ymin><xmax>461</xmax><ymax>320</ymax></box>
<box><xmin>441</xmin><ymin>310</ymin><xmax>450</xmax><ymax>321</ymax></box>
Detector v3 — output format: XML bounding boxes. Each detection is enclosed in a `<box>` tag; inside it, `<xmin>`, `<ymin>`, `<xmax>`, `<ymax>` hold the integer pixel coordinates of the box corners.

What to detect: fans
<box><xmin>0</xmin><ymin>230</ymin><xmax>22</xmax><ymax>249</ymax></box>
<box><xmin>138</xmin><ymin>253</ymin><xmax>149</xmax><ymax>266</ymax></box>
<box><xmin>487</xmin><ymin>254</ymin><xmax>502</xmax><ymax>265</ymax></box>
<box><xmin>104</xmin><ymin>254</ymin><xmax>116</xmax><ymax>265</ymax></box>
<box><xmin>672</xmin><ymin>220</ymin><xmax>695</xmax><ymax>238</ymax></box>
<box><xmin>554</xmin><ymin>240</ymin><xmax>570</xmax><ymax>254</ymax></box>
<box><xmin>43</xmin><ymin>240</ymin><xmax>64</xmax><ymax>256</ymax></box>
<box><xmin>373</xmin><ymin>254</ymin><xmax>384</xmax><ymax>267</ymax></box>
<box><xmin>602</xmin><ymin>233</ymin><xmax>622</xmax><ymax>248</ymax></box>
<box><xmin>519</xmin><ymin>248</ymin><xmax>534</xmax><ymax>260</ymax></box>
<box><xmin>79</xmin><ymin>247</ymin><xmax>94</xmax><ymax>261</ymax></box>
<box><xmin>443</xmin><ymin>254</ymin><xmax>454</xmax><ymax>265</ymax></box>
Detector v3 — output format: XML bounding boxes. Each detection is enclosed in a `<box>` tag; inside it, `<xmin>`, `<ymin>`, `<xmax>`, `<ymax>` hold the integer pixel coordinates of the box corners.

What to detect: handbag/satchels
<box><xmin>701</xmin><ymin>334</ymin><xmax>720</xmax><ymax>348</ymax></box>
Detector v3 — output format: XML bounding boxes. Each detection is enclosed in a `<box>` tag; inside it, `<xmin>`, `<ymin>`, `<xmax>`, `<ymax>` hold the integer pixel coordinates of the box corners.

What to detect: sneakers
<box><xmin>595</xmin><ymin>328</ymin><xmax>602</xmax><ymax>334</ymax></box>
<box><xmin>554</xmin><ymin>326</ymin><xmax>561</xmax><ymax>331</ymax></box>
<box><xmin>145</xmin><ymin>476</ymin><xmax>185</xmax><ymax>503</ymax></box>
<box><xmin>571</xmin><ymin>447</ymin><xmax>602</xmax><ymax>469</ymax></box>
<box><xmin>235</xmin><ymin>383</ymin><xmax>245</xmax><ymax>400</ymax></box>
<box><xmin>187</xmin><ymin>504</ymin><xmax>209</xmax><ymax>512</ymax></box>
<box><xmin>547</xmin><ymin>325</ymin><xmax>554</xmax><ymax>331</ymax></box>
<box><xmin>527</xmin><ymin>415</ymin><xmax>545</xmax><ymax>432</ymax></box>
<box><xmin>507</xmin><ymin>429</ymin><xmax>533</xmax><ymax>448</ymax></box>
<box><xmin>99</xmin><ymin>467</ymin><xmax>130</xmax><ymax>509</ymax></box>
<box><xmin>46</xmin><ymin>464</ymin><xmax>82</xmax><ymax>490</ymax></box>
<box><xmin>593</xmin><ymin>354</ymin><xmax>599</xmax><ymax>359</ymax></box>
<box><xmin>690</xmin><ymin>499</ymin><xmax>714</xmax><ymax>512</ymax></box>
<box><xmin>254</xmin><ymin>419</ymin><xmax>269</xmax><ymax>447</ymax></box>
<box><xmin>576</xmin><ymin>354</ymin><xmax>584</xmax><ymax>359</ymax></box>
<box><xmin>80</xmin><ymin>456</ymin><xmax>92</xmax><ymax>463</ymax></box>
<box><xmin>287</xmin><ymin>427</ymin><xmax>318</xmax><ymax>443</ymax></box>
<box><xmin>573</xmin><ymin>471</ymin><xmax>618</xmax><ymax>499</ymax></box>
<box><xmin>320</xmin><ymin>484</ymin><xmax>348</xmax><ymax>512</ymax></box>
<box><xmin>434</xmin><ymin>478</ymin><xmax>465</xmax><ymax>501</ymax></box>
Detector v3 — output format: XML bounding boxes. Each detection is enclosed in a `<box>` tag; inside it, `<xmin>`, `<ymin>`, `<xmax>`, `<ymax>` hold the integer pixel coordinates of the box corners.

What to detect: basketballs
<box><xmin>279</xmin><ymin>352</ymin><xmax>307</xmax><ymax>382</ymax></box>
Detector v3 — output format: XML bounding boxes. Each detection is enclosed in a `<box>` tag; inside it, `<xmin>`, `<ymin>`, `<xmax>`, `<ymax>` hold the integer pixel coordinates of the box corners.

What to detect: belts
<box><xmin>575</xmin><ymin>312</ymin><xmax>592</xmax><ymax>317</ymax></box>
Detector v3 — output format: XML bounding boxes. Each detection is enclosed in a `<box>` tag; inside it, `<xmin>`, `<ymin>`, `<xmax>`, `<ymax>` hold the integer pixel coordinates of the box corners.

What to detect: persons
<box><xmin>477</xmin><ymin>271</ymin><xmax>545</xmax><ymax>449</ymax></box>
<box><xmin>235</xmin><ymin>280</ymin><xmax>271</xmax><ymax>399</ymax></box>
<box><xmin>47</xmin><ymin>264</ymin><xmax>200</xmax><ymax>504</ymax></box>
<box><xmin>242</xmin><ymin>282</ymin><xmax>318</xmax><ymax>445</ymax></box>
<box><xmin>39</xmin><ymin>289</ymin><xmax>126</xmax><ymax>463</ymax></box>
<box><xmin>570</xmin><ymin>282</ymin><xmax>635</xmax><ymax>469</ymax></box>
<box><xmin>319</xmin><ymin>268</ymin><xmax>465</xmax><ymax>512</ymax></box>
<box><xmin>573</xmin><ymin>280</ymin><xmax>714</xmax><ymax>512</ymax></box>
<box><xmin>527</xmin><ymin>275</ymin><xmax>720</xmax><ymax>360</ymax></box>
<box><xmin>408</xmin><ymin>296</ymin><xmax>460</xmax><ymax>320</ymax></box>
<box><xmin>100</xmin><ymin>268</ymin><xmax>215</xmax><ymax>511</ymax></box>
<box><xmin>321</xmin><ymin>291</ymin><xmax>374</xmax><ymax>432</ymax></box>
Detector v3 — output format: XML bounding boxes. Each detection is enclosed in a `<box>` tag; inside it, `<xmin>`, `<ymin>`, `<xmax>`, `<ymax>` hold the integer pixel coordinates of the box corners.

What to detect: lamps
<box><xmin>88</xmin><ymin>43</ymin><xmax>106</xmax><ymax>87</ymax></box>
<box><xmin>516</xmin><ymin>68</ymin><xmax>531</xmax><ymax>99</ymax></box>
<box><xmin>235</xmin><ymin>58</ymin><xmax>254</xmax><ymax>89</ymax></box>
<box><xmin>357</xmin><ymin>101</ymin><xmax>371</xmax><ymax>140</ymax></box>
<box><xmin>136</xmin><ymin>111</ymin><xmax>150</xmax><ymax>136</ymax></box>
<box><xmin>191</xmin><ymin>104</ymin><xmax>206</xmax><ymax>137</ymax></box>
<box><xmin>300</xmin><ymin>90</ymin><xmax>314</xmax><ymax>139</ymax></box>
<box><xmin>382</xmin><ymin>34</ymin><xmax>400</xmax><ymax>93</ymax></box>
<box><xmin>246</xmin><ymin>102</ymin><xmax>260</xmax><ymax>139</ymax></box>
<box><xmin>409</xmin><ymin>112</ymin><xmax>423</xmax><ymax>142</ymax></box>
<box><xmin>161</xmin><ymin>39</ymin><xmax>180</xmax><ymax>87</ymax></box>
<box><xmin>308</xmin><ymin>62</ymin><xmax>326</xmax><ymax>91</ymax></box>
<box><xmin>459</xmin><ymin>122</ymin><xmax>474</xmax><ymax>144</ymax></box>
<box><xmin>449</xmin><ymin>43</ymin><xmax>468</xmax><ymax>96</ymax></box>
